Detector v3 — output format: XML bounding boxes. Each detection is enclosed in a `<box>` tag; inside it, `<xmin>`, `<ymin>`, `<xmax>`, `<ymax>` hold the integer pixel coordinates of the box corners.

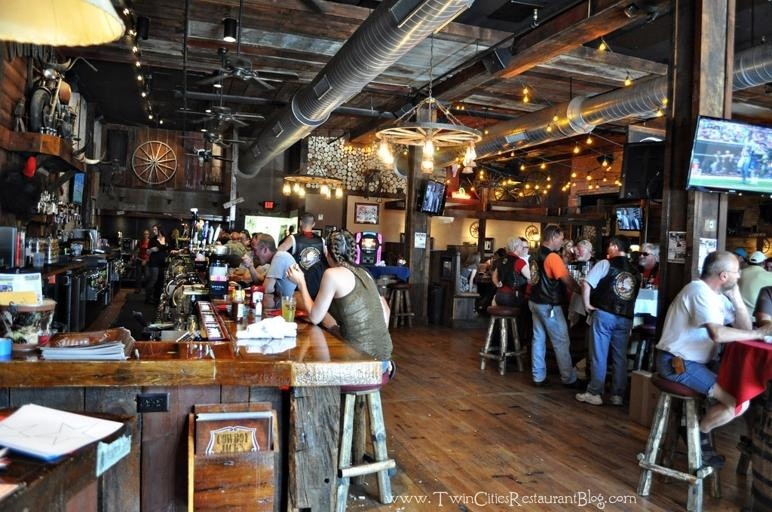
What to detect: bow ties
<box><xmin>575</xmin><ymin>392</ymin><xmax>604</xmax><ymax>405</ymax></box>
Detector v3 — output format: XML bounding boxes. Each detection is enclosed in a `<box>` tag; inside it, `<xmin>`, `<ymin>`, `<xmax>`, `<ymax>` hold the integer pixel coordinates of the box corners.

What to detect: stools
<box><xmin>624</xmin><ymin>324</ymin><xmax>656</xmax><ymax>373</ymax></box>
<box><xmin>480</xmin><ymin>306</ymin><xmax>525</xmax><ymax>376</ymax></box>
<box><xmin>336</xmin><ymin>370</ymin><xmax>393</xmax><ymax>512</ymax></box>
<box><xmin>388</xmin><ymin>282</ymin><xmax>415</xmax><ymax>328</ymax></box>
<box><xmin>637</xmin><ymin>373</ymin><xmax>725</xmax><ymax>512</ymax></box>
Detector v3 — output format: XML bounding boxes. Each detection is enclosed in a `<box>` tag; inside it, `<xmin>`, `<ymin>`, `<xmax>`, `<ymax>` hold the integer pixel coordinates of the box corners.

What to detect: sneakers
<box><xmin>609</xmin><ymin>394</ymin><xmax>623</xmax><ymax>405</ymax></box>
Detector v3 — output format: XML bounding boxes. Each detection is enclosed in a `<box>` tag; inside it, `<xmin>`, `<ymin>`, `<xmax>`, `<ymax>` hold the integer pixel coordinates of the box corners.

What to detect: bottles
<box><xmin>226</xmin><ymin>286</ymin><xmax>235</xmax><ymax>315</ymax></box>
<box><xmin>255</xmin><ymin>298</ymin><xmax>263</xmax><ymax>319</ymax></box>
<box><xmin>250</xmin><ymin>280</ymin><xmax>264</xmax><ymax>311</ymax></box>
<box><xmin>231</xmin><ymin>285</ymin><xmax>246</xmax><ymax>323</ymax></box>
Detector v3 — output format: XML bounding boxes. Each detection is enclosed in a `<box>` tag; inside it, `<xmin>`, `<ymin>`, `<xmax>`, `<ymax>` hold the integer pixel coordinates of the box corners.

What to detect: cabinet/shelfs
<box><xmin>82</xmin><ymin>257</ymin><xmax>127</xmax><ymax>325</ymax></box>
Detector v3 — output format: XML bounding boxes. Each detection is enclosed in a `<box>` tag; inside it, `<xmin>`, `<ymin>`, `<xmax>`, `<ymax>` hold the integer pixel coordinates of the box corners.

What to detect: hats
<box><xmin>749</xmin><ymin>251</ymin><xmax>768</xmax><ymax>263</ymax></box>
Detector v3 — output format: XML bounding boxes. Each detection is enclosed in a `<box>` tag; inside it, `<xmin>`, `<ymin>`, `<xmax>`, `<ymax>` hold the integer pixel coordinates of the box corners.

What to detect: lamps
<box><xmin>222</xmin><ymin>17</ymin><xmax>239</xmax><ymax>44</ymax></box>
<box><xmin>0</xmin><ymin>1</ymin><xmax>126</xmax><ymax>47</ymax></box>
<box><xmin>118</xmin><ymin>8</ymin><xmax>162</xmax><ymax>123</ymax></box>
<box><xmin>281</xmin><ymin>128</ymin><xmax>344</xmax><ymax>202</ymax></box>
<box><xmin>374</xmin><ymin>33</ymin><xmax>485</xmax><ymax>172</ymax></box>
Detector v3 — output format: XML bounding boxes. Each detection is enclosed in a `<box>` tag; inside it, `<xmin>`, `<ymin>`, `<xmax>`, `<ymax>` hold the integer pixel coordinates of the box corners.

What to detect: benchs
<box><xmin>447</xmin><ymin>248</ymin><xmax>480</xmax><ymax>319</ymax></box>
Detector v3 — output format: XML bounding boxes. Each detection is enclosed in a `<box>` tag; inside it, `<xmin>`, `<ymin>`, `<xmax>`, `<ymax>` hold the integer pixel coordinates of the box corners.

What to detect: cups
<box><xmin>33</xmin><ymin>253</ymin><xmax>45</xmax><ymax>266</ymax></box>
<box><xmin>279</xmin><ymin>296</ymin><xmax>297</xmax><ymax>321</ymax></box>
<box><xmin>0</xmin><ymin>338</ymin><xmax>13</xmax><ymax>357</ymax></box>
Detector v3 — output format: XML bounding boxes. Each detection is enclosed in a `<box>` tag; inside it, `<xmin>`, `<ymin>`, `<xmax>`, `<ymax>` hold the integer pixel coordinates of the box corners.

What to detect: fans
<box><xmin>183</xmin><ymin>150</ymin><xmax>233</xmax><ymax>165</ymax></box>
<box><xmin>178</xmin><ymin>85</ymin><xmax>268</xmax><ymax>128</ymax></box>
<box><xmin>197</xmin><ymin>58</ymin><xmax>299</xmax><ymax>91</ymax></box>
<box><xmin>178</xmin><ymin>121</ymin><xmax>249</xmax><ymax>147</ymax></box>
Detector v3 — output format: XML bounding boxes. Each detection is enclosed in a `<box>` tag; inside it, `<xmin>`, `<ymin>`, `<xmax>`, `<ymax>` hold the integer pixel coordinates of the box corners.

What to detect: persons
<box><xmin>737</xmin><ymin>251</ymin><xmax>772</xmax><ymax>321</ymax></box>
<box><xmin>753</xmin><ymin>285</ymin><xmax>772</xmax><ymax>326</ymax></box>
<box><xmin>690</xmin><ymin>118</ymin><xmax>772</xmax><ymax>194</ymax></box>
<box><xmin>654</xmin><ymin>250</ymin><xmax>771</xmax><ymax>466</ymax></box>
<box><xmin>460</xmin><ymin>224</ymin><xmax>662</xmax><ymax>408</ymax></box>
<box><xmin>619</xmin><ymin>208</ymin><xmax>631</xmax><ymax>230</ymax></box>
<box><xmin>765</xmin><ymin>257</ymin><xmax>772</xmax><ymax>271</ymax></box>
<box><xmin>423</xmin><ymin>182</ymin><xmax>444</xmax><ymax>212</ymax></box>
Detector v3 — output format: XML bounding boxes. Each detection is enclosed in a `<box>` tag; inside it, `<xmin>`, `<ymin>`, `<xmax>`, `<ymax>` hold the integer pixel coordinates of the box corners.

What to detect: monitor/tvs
<box><xmin>619</xmin><ymin>124</ymin><xmax>666</xmax><ymax>199</ymax></box>
<box><xmin>684</xmin><ymin>114</ymin><xmax>772</xmax><ymax>200</ymax></box>
<box><xmin>615</xmin><ymin>207</ymin><xmax>643</xmax><ymax>237</ymax></box>
<box><xmin>417</xmin><ymin>179</ymin><xmax>448</xmax><ymax>217</ymax></box>
<box><xmin>69</xmin><ymin>173</ymin><xmax>86</xmax><ymax>206</ymax></box>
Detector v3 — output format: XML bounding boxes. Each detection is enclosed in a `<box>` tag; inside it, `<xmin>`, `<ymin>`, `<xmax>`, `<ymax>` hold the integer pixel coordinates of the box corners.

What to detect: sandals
<box><xmin>680</xmin><ymin>426</ymin><xmax>712</xmax><ymax>465</ymax></box>
<box><xmin>713</xmin><ymin>451</ymin><xmax>726</xmax><ymax>469</ymax></box>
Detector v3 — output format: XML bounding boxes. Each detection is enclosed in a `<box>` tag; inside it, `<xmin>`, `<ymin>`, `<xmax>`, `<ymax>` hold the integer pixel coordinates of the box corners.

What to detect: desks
<box><xmin>0</xmin><ymin>403</ymin><xmax>138</xmax><ymax>512</ymax></box>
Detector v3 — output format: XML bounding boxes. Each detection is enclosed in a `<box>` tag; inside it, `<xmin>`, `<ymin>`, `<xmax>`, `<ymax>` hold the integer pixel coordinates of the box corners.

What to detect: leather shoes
<box><xmin>563</xmin><ymin>379</ymin><xmax>585</xmax><ymax>390</ymax></box>
<box><xmin>534</xmin><ymin>378</ymin><xmax>550</xmax><ymax>386</ymax></box>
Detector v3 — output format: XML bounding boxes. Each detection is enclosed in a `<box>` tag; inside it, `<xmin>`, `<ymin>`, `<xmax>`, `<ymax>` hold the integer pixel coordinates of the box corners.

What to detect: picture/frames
<box><xmin>355</xmin><ymin>202</ymin><xmax>379</xmax><ymax>224</ymax></box>
<box><xmin>485</xmin><ymin>238</ymin><xmax>494</xmax><ymax>253</ymax></box>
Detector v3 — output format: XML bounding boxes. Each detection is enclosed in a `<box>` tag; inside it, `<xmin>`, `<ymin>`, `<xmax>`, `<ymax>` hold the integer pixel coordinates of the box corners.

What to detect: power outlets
<box><xmin>137</xmin><ymin>393</ymin><xmax>166</xmax><ymax>412</ymax></box>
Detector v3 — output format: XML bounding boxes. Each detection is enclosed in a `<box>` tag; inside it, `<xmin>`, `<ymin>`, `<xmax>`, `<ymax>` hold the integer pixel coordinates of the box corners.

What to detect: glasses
<box><xmin>718</xmin><ymin>270</ymin><xmax>743</xmax><ymax>275</ymax></box>
<box><xmin>636</xmin><ymin>252</ymin><xmax>653</xmax><ymax>257</ymax></box>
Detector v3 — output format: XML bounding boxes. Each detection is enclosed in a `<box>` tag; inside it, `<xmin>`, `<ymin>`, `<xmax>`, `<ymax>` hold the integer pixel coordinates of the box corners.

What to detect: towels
<box><xmin>235</xmin><ymin>315</ymin><xmax>298</xmax><ymax>339</ymax></box>
<box><xmin>236</xmin><ymin>340</ymin><xmax>296</xmax><ymax>355</ymax></box>
